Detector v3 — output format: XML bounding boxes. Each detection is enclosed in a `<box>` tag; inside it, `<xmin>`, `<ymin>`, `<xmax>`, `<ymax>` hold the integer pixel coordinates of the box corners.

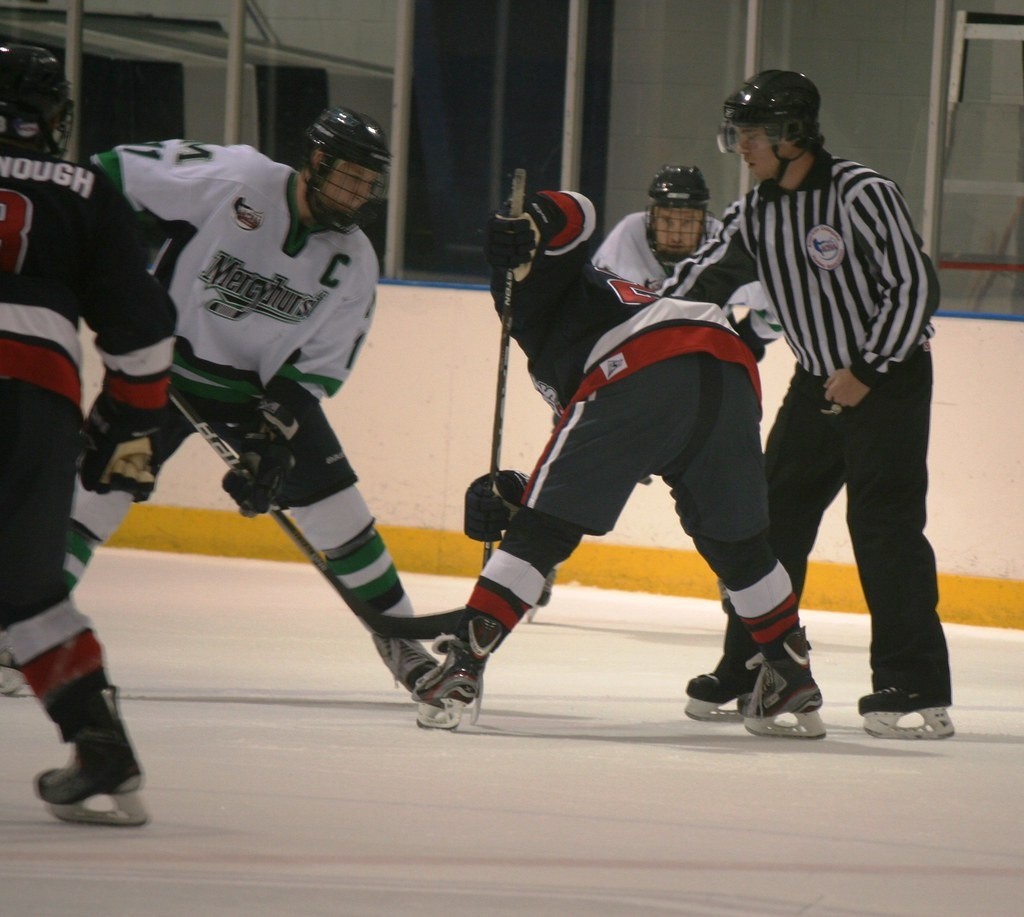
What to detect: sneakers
<box><xmin>735</xmin><ymin>625</ymin><xmax>826</xmax><ymax>740</ymax></box>
<box><xmin>411</xmin><ymin>608</ymin><xmax>505</xmax><ymax>722</ymax></box>
<box><xmin>685</xmin><ymin>656</ymin><xmax>759</xmax><ymax>721</ymax></box>
<box><xmin>526</xmin><ymin>568</ymin><xmax>556</xmax><ymax>621</ymax></box>
<box><xmin>857</xmin><ymin>684</ymin><xmax>954</xmax><ymax>740</ymax></box>
<box><xmin>32</xmin><ymin>687</ymin><xmax>150</xmax><ymax>827</ymax></box>
<box><xmin>373</xmin><ymin>631</ymin><xmax>484</xmax><ymax>729</ymax></box>
<box><xmin>0</xmin><ymin>645</ymin><xmax>26</xmax><ymax>693</ymax></box>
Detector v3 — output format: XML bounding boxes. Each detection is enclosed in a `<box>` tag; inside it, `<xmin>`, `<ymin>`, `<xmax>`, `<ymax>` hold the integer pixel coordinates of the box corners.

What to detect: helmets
<box><xmin>645</xmin><ymin>165</ymin><xmax>710</xmax><ymax>263</ymax></box>
<box><xmin>722</xmin><ymin>70</ymin><xmax>820</xmax><ymax>143</ymax></box>
<box><xmin>0</xmin><ymin>46</ymin><xmax>74</xmax><ymax>159</ymax></box>
<box><xmin>305</xmin><ymin>106</ymin><xmax>392</xmax><ymax>235</ymax></box>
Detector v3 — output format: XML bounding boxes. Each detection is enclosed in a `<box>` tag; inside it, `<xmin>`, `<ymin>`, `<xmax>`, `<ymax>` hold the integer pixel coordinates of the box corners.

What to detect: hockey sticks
<box><xmin>468</xmin><ymin>163</ymin><xmax>532</xmax><ymax>727</ymax></box>
<box><xmin>167</xmin><ymin>379</ymin><xmax>471</xmax><ymax>641</ymax></box>
<box><xmin>75</xmin><ymin>449</ymin><xmax>150</xmax><ymax>472</ymax></box>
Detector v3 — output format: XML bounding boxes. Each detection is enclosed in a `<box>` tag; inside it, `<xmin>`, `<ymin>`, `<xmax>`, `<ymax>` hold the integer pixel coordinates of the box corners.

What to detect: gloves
<box><xmin>223</xmin><ymin>403</ymin><xmax>300</xmax><ymax>517</ymax></box>
<box><xmin>735</xmin><ymin>316</ymin><xmax>766</xmax><ymax>362</ymax></box>
<box><xmin>464</xmin><ymin>467</ymin><xmax>531</xmax><ymax>542</ymax></box>
<box><xmin>79</xmin><ymin>391</ymin><xmax>164</xmax><ymax>504</ymax></box>
<box><xmin>488</xmin><ymin>193</ymin><xmax>555</xmax><ymax>268</ymax></box>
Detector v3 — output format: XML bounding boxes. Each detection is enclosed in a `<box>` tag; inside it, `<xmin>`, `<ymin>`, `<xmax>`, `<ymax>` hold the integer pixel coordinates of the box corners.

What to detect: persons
<box><xmin>413</xmin><ymin>190</ymin><xmax>828</xmax><ymax>740</ymax></box>
<box><xmin>656</xmin><ymin>68</ymin><xmax>953</xmax><ymax>741</ymax></box>
<box><xmin>0</xmin><ymin>42</ymin><xmax>179</xmax><ymax>824</ymax></box>
<box><xmin>0</xmin><ymin>105</ymin><xmax>438</xmax><ymax>692</ymax></box>
<box><xmin>592</xmin><ymin>165</ymin><xmax>783</xmax><ymax>362</ymax></box>
